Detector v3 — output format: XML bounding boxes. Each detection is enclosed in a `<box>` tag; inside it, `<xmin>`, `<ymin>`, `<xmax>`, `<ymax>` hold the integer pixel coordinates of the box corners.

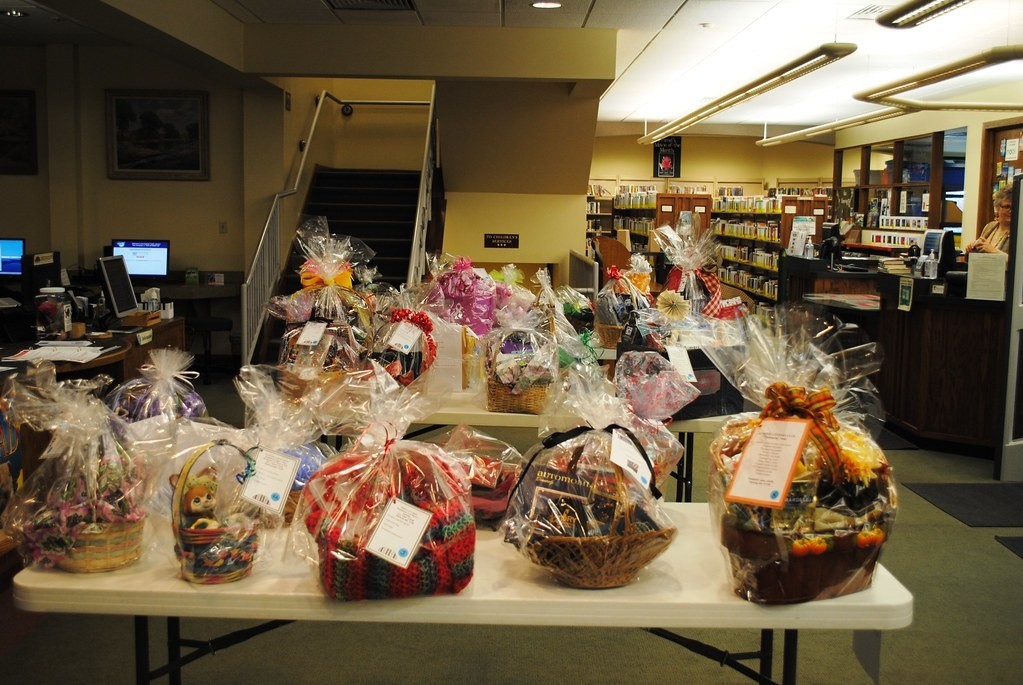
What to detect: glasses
<box><xmin>1000</xmin><ymin>204</ymin><xmax>1012</xmax><ymax>208</ymax></box>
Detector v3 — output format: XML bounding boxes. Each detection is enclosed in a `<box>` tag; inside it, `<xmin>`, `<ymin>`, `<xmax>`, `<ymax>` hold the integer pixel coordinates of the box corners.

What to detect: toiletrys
<box><xmin>804</xmin><ymin>236</ymin><xmax>814</xmax><ymax>258</ymax></box>
<box><xmin>924</xmin><ymin>248</ymin><xmax>938</xmax><ymax>278</ymax></box>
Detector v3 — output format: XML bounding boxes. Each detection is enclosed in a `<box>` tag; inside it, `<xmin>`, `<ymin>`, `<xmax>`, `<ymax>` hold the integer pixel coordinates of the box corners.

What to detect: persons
<box><xmin>966</xmin><ymin>183</ymin><xmax>1014</xmax><ymax>264</ymax></box>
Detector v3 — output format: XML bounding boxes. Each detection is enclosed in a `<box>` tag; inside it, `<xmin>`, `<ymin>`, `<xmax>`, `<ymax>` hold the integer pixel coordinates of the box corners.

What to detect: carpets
<box><xmin>900</xmin><ymin>481</ymin><xmax>1023</xmax><ymax>528</ymax></box>
<box><xmin>994</xmin><ymin>535</ymin><xmax>1023</xmax><ymax>558</ymax></box>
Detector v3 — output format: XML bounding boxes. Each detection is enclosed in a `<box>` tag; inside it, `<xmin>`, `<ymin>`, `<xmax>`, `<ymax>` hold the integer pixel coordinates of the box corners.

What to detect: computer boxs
<box><xmin>21</xmin><ymin>250</ymin><xmax>61</xmax><ymax>294</ymax></box>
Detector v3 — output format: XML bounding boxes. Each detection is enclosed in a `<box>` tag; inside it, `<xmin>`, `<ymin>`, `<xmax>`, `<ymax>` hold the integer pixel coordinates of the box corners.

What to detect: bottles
<box><xmin>881</xmin><ymin>168</ymin><xmax>889</xmax><ymax>185</ymax></box>
<box><xmin>99</xmin><ymin>290</ymin><xmax>105</xmax><ymax>316</ymax></box>
<box><xmin>34</xmin><ymin>286</ymin><xmax>72</xmax><ymax>332</ymax></box>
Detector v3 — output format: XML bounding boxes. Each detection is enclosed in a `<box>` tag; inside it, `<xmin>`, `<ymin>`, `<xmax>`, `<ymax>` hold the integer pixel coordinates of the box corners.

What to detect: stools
<box><xmin>180</xmin><ymin>316</ymin><xmax>232</xmax><ymax>385</ymax></box>
<box><xmin>802</xmin><ymin>292</ymin><xmax>885</xmax><ymax>411</ymax></box>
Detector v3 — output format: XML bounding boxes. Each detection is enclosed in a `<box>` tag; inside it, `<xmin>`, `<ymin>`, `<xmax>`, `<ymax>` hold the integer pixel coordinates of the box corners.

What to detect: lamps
<box><xmin>637</xmin><ymin>0</ymin><xmax>1023</xmax><ymax>148</ymax></box>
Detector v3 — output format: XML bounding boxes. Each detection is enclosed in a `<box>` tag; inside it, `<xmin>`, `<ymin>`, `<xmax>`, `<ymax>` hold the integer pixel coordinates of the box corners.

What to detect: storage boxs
<box><xmin>616</xmin><ymin>330</ymin><xmax>765</xmax><ymax>419</ymax></box>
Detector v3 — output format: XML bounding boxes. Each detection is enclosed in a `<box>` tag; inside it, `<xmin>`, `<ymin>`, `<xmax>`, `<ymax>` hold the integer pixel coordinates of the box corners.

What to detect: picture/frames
<box><xmin>104</xmin><ymin>87</ymin><xmax>214</xmax><ymax>181</ymax></box>
<box><xmin>0</xmin><ymin>88</ymin><xmax>40</xmax><ymax>175</ymax></box>
<box><xmin>283</xmin><ymin>92</ymin><xmax>294</xmax><ymax>110</ymax></box>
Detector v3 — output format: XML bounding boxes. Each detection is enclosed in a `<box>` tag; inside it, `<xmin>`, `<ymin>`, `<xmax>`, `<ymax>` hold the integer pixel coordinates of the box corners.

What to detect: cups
<box><xmin>902</xmin><ymin>168</ymin><xmax>910</xmax><ymax>183</ymax></box>
<box><xmin>137</xmin><ymin>298</ymin><xmax>159</xmax><ymax>311</ymax></box>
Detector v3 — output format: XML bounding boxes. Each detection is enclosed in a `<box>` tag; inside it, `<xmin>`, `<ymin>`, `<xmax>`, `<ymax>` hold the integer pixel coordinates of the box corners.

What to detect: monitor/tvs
<box><xmin>920</xmin><ymin>229</ymin><xmax>956</xmax><ymax>269</ymax></box>
<box><xmin>112</xmin><ymin>238</ymin><xmax>170</xmax><ymax>280</ymax></box>
<box><xmin>821</xmin><ymin>223</ymin><xmax>842</xmax><ymax>264</ymax></box>
<box><xmin>96</xmin><ymin>255</ymin><xmax>139</xmax><ymax>317</ymax></box>
<box><xmin>0</xmin><ymin>238</ymin><xmax>26</xmax><ymax>277</ymax></box>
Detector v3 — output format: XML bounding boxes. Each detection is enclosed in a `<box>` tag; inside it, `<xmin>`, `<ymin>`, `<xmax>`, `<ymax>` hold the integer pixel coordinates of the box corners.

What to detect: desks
<box><xmin>11</xmin><ymin>499</ymin><xmax>912</xmax><ymax>685</ymax></box>
<box><xmin>589</xmin><ymin>348</ymin><xmax>619</xmax><ymax>375</ymax></box>
<box><xmin>305</xmin><ymin>405</ymin><xmax>768</xmax><ymax>504</ymax></box>
<box><xmin>99</xmin><ymin>278</ymin><xmax>241</xmax><ymax>386</ymax></box>
<box><xmin>0</xmin><ymin>327</ymin><xmax>131</xmax><ymax>483</ymax></box>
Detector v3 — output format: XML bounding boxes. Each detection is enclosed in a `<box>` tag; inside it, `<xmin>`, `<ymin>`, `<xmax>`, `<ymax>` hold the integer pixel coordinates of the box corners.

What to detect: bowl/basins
<box><xmin>853</xmin><ymin>169</ymin><xmax>892</xmax><ymax>185</ymax></box>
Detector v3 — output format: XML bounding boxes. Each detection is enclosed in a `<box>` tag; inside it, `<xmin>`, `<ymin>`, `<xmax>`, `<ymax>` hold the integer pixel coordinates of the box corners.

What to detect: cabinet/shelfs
<box><xmin>85</xmin><ymin>312</ymin><xmax>187</xmax><ymax>383</ymax></box>
<box><xmin>585</xmin><ymin>196</ymin><xmax>617</xmax><ymax>260</ymax></box>
<box><xmin>832</xmin><ymin>182</ymin><xmax>931</xmax><ymax>252</ymax></box>
<box><xmin>711</xmin><ymin>192</ymin><xmax>829</xmax><ymax>301</ymax></box>
<box><xmin>612</xmin><ymin>194</ymin><xmax>714</xmax><ymax>302</ymax></box>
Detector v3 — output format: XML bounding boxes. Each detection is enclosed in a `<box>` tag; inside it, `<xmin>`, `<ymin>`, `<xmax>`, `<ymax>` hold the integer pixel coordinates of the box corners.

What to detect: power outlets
<box><xmin>219</xmin><ymin>219</ymin><xmax>226</xmax><ymax>235</ymax></box>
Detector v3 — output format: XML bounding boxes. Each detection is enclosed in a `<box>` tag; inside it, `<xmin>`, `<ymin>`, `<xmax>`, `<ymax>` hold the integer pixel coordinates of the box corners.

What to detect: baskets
<box><xmin>519</xmin><ymin>443</ymin><xmax>678</xmax><ymax>590</ymax></box>
<box><xmin>313</xmin><ymin>458</ymin><xmax>472</xmax><ymax>600</ymax></box>
<box><xmin>470</xmin><ymin>472</ymin><xmax>513</xmax><ymax>520</ymax></box>
<box><xmin>43</xmin><ymin>514</ymin><xmax>146</xmax><ymax>573</ymax></box>
<box><xmin>171</xmin><ymin>440</ymin><xmax>262</xmax><ymax>584</ymax></box>
<box><xmin>715</xmin><ymin>516</ymin><xmax>886</xmax><ymax>606</ymax></box>
<box><xmin>486</xmin><ymin>277</ymin><xmax>638</xmax><ymax>414</ymax></box>
<box><xmin>257</xmin><ymin>485</ymin><xmax>309</xmax><ymax>532</ymax></box>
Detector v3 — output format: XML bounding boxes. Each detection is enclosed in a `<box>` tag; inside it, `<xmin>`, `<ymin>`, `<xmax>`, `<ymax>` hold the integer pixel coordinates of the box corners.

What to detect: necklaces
<box><xmin>989</xmin><ymin>227</ymin><xmax>1008</xmax><ymax>248</ymax></box>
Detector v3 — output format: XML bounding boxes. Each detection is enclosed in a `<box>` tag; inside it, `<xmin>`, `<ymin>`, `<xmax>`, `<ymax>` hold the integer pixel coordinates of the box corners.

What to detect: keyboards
<box><xmin>839</xmin><ymin>264</ymin><xmax>868</xmax><ymax>272</ymax></box>
